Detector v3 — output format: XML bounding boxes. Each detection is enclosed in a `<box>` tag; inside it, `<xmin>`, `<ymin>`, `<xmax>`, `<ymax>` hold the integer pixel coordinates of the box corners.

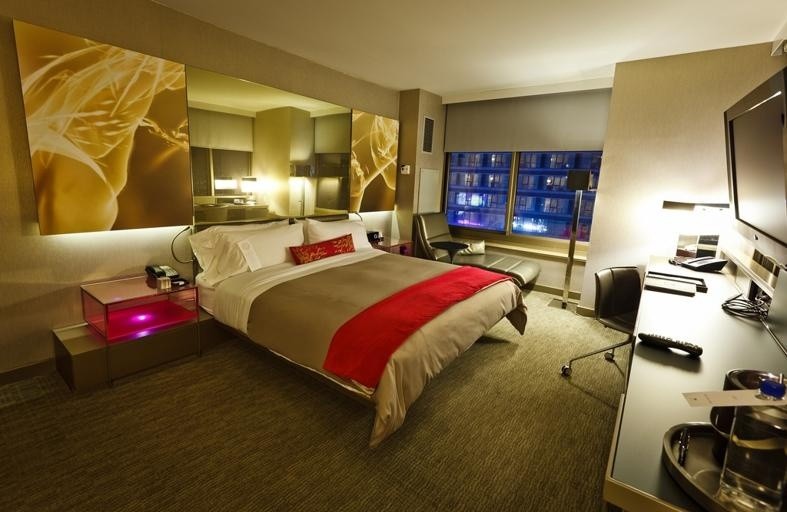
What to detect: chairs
<box><xmin>410</xmin><ymin>213</ymin><xmax>541</xmax><ymax>293</ymax></box>
<box><xmin>559</xmin><ymin>265</ymin><xmax>642</xmax><ymax>378</ymax></box>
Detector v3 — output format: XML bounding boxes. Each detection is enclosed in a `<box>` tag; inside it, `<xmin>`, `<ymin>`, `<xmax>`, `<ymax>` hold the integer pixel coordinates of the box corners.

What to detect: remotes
<box><xmin>638</xmin><ymin>332</ymin><xmax>703</xmax><ymax>357</ymax></box>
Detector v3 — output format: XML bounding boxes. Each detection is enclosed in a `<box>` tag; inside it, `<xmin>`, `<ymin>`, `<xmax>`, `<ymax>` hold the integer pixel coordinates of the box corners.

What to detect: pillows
<box><xmin>293</xmin><ymin>216</ymin><xmax>363</xmax><ymax>245</ymax></box>
<box><xmin>305</xmin><ymin>220</ymin><xmax>373</xmax><ymax>254</ymax></box>
<box><xmin>287</xmin><ymin>233</ymin><xmax>356</xmax><ymax>266</ymax></box>
<box><xmin>234</xmin><ymin>222</ymin><xmax>305</xmax><ymax>273</ymax></box>
<box><xmin>458</xmin><ymin>239</ymin><xmax>486</xmax><ymax>256</ymax></box>
<box><xmin>188</xmin><ymin>218</ymin><xmax>290</xmax><ymax>285</ymax></box>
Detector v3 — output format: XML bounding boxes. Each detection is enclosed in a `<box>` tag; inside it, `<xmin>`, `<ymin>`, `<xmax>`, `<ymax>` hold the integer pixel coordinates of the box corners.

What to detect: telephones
<box><xmin>145</xmin><ymin>264</ymin><xmax>180</xmax><ymax>280</ymax></box>
<box><xmin>681</xmin><ymin>255</ymin><xmax>728</xmax><ymax>272</ymax></box>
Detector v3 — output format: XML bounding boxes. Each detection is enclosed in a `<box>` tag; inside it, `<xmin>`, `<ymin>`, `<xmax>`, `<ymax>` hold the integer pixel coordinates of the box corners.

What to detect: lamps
<box><xmin>545</xmin><ymin>167</ymin><xmax>592</xmax><ymax>314</ymax></box>
<box><xmin>289</xmin><ymin>164</ymin><xmax>316</xmax><ymax>215</ymax></box>
<box><xmin>212</xmin><ymin>178</ymin><xmax>236</xmax><ymax>190</ymax></box>
<box><xmin>240</xmin><ymin>176</ymin><xmax>258</xmax><ymax>198</ymax></box>
<box><xmin>661</xmin><ymin>196</ymin><xmax>732</xmax><ymax>216</ymax></box>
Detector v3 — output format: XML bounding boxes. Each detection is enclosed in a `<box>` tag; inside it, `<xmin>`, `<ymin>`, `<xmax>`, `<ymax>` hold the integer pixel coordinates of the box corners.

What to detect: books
<box><xmin>644</xmin><ymin>275</ymin><xmax>696</xmax><ymax>296</ymax></box>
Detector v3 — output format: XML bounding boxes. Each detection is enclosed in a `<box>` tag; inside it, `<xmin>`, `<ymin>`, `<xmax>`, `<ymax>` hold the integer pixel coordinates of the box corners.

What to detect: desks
<box><xmin>194</xmin><ymin>204</ymin><xmax>268</xmax><ymax>222</ymax></box>
<box><xmin>429</xmin><ymin>241</ymin><xmax>468</xmax><ymax>263</ymax></box>
<box><xmin>602</xmin><ymin>250</ymin><xmax>787</xmax><ymax>510</ymax></box>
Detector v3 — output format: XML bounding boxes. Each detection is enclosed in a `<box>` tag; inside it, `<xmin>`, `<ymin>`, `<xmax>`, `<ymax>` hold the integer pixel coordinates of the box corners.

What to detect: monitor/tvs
<box><xmin>724</xmin><ymin>66</ymin><xmax>787</xmax><ymax>273</ymax></box>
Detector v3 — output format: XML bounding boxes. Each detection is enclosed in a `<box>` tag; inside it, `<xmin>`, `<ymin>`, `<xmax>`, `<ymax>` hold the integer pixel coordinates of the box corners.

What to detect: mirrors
<box><xmin>185</xmin><ymin>62</ymin><xmax>351</xmax><ymax>226</ymax></box>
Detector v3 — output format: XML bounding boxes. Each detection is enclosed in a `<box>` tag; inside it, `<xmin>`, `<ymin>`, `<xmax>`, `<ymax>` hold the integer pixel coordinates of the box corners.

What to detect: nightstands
<box><xmin>78</xmin><ymin>272</ymin><xmax>206</xmax><ymax>394</ymax></box>
<box><xmin>374</xmin><ymin>237</ymin><xmax>412</xmax><ymax>256</ymax></box>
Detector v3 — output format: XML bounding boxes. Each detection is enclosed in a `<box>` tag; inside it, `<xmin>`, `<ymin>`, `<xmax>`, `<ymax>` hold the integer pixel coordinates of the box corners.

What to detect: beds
<box><xmin>188</xmin><ymin>211</ymin><xmax>528</xmax><ymax>450</ymax></box>
